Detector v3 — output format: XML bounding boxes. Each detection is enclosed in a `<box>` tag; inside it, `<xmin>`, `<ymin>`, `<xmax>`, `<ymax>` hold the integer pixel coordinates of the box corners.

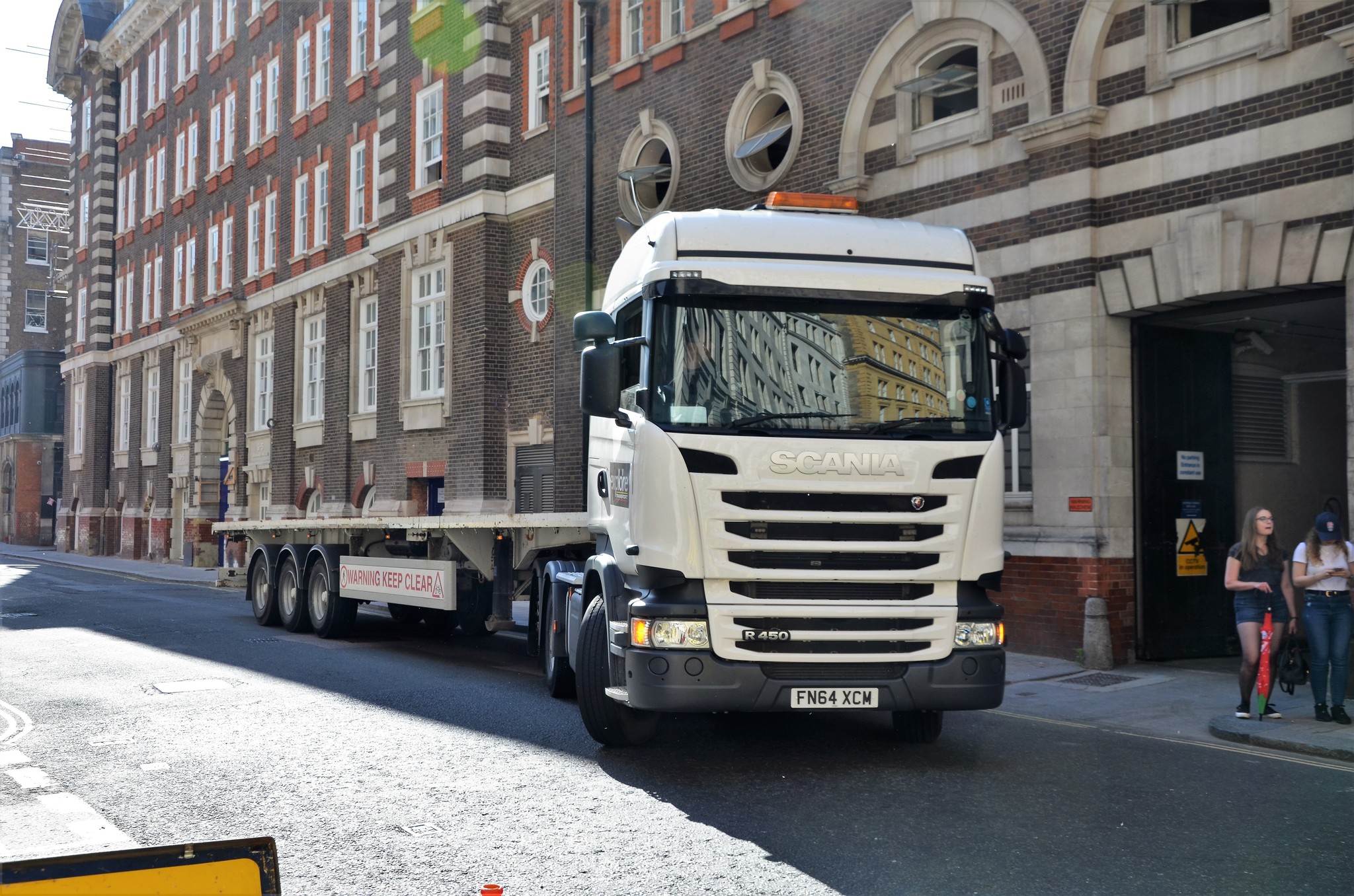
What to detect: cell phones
<box><xmin>1333</xmin><ymin>567</ymin><xmax>1344</xmax><ymax>571</ymax></box>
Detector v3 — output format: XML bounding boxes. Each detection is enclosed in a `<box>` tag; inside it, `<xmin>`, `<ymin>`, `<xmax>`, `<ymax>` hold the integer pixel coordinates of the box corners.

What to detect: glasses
<box><xmin>1255</xmin><ymin>517</ymin><xmax>1274</xmax><ymax>521</ymax></box>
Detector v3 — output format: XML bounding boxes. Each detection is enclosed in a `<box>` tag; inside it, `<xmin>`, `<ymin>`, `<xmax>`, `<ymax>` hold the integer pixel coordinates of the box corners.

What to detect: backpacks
<box><xmin>1273</xmin><ymin>633</ymin><xmax>1310</xmax><ymax>696</ymax></box>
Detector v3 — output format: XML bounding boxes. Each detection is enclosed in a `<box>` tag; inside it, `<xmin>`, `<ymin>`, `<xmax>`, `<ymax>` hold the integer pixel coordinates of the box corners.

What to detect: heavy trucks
<box><xmin>209</xmin><ymin>193</ymin><xmax>1028</xmax><ymax>754</ymax></box>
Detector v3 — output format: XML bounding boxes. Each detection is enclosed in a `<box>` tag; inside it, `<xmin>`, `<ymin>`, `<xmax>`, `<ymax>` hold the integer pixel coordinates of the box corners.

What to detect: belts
<box><xmin>1304</xmin><ymin>589</ymin><xmax>1350</xmax><ymax>597</ymax></box>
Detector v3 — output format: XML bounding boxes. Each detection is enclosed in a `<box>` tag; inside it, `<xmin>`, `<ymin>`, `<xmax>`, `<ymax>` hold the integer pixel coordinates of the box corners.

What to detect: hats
<box><xmin>1315</xmin><ymin>512</ymin><xmax>1343</xmax><ymax>541</ymax></box>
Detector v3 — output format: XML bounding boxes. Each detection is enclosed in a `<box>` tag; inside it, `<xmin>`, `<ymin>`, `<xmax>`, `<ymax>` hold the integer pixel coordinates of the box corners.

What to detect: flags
<box><xmin>47</xmin><ymin>498</ymin><xmax>54</xmax><ymax>506</ymax></box>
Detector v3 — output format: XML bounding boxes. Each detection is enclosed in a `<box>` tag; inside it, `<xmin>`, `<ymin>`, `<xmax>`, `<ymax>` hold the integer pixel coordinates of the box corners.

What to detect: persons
<box><xmin>1224</xmin><ymin>507</ymin><xmax>1297</xmax><ymax>718</ymax></box>
<box><xmin>1291</xmin><ymin>512</ymin><xmax>1354</xmax><ymax>725</ymax></box>
<box><xmin>661</xmin><ymin>330</ymin><xmax>727</xmax><ymax>395</ymax></box>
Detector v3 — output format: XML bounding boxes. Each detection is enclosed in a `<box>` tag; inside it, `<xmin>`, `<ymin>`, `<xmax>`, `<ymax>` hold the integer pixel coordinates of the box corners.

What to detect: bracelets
<box><xmin>1348</xmin><ymin>574</ymin><xmax>1353</xmax><ymax>579</ymax></box>
<box><xmin>1290</xmin><ymin>617</ymin><xmax>1298</xmax><ymax>619</ymax></box>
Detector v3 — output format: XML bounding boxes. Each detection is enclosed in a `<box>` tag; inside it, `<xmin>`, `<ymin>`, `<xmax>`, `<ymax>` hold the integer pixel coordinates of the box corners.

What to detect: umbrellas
<box><xmin>1258</xmin><ymin>592</ymin><xmax>1274</xmax><ymax>720</ymax></box>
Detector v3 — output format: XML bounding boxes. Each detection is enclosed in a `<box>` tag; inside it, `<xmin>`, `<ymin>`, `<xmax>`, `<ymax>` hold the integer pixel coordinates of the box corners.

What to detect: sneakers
<box><xmin>1235</xmin><ymin>703</ymin><xmax>1251</xmax><ymax>719</ymax></box>
<box><xmin>1330</xmin><ymin>705</ymin><xmax>1351</xmax><ymax>725</ymax></box>
<box><xmin>1259</xmin><ymin>703</ymin><xmax>1282</xmax><ymax>719</ymax></box>
<box><xmin>1316</xmin><ymin>703</ymin><xmax>1331</xmax><ymax>722</ymax></box>
